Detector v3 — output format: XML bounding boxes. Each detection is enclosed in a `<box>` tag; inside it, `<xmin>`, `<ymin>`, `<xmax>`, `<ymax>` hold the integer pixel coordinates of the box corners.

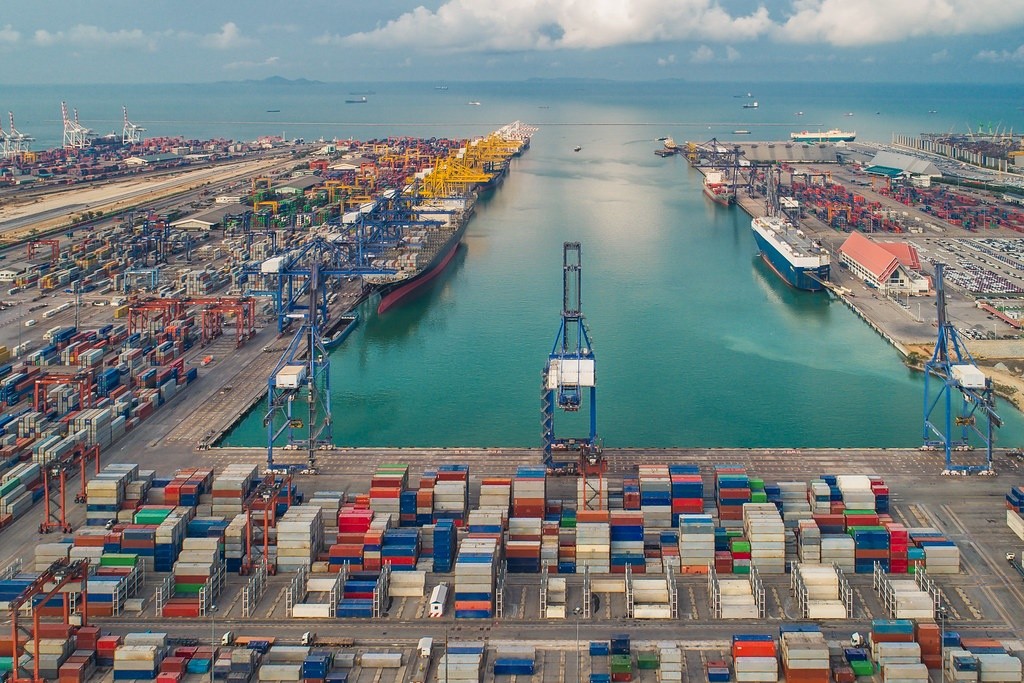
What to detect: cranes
<box><xmin>263</xmin><ymin>255</ymin><xmax>333</xmax><ymax>475</ymax></box>
<box><xmin>275</xmin><ymin>129</ymin><xmax>523</xmax><ymax>334</ymax></box>
<box><xmin>123</xmin><ymin>105</ymin><xmax>148</xmax><ymax>144</ymax></box>
<box><xmin>0</xmin><ymin>111</ymin><xmax>36</xmax><ymax>157</ymax></box>
<box><xmin>924</xmin><ymin>260</ymin><xmax>1003</xmax><ymax>474</ymax></box>
<box><xmin>541</xmin><ymin>240</ymin><xmax>596</xmax><ymax>475</ymax></box>
<box><xmin>61</xmin><ymin>100</ymin><xmax>93</xmax><ymax>149</ymax></box>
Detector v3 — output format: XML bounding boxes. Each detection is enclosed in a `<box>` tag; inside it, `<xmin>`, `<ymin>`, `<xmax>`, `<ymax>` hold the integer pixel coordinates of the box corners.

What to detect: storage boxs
<box><xmin>0</xmin><ymin>134</ymin><xmax>1024</xmax><ymax>683</ymax></box>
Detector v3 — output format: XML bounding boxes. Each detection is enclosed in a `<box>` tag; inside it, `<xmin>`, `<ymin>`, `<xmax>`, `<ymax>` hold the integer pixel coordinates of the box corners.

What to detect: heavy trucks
<box><xmin>221</xmin><ymin>631</ymin><xmax>276</xmax><ymax>647</ymax></box>
<box><xmin>301</xmin><ymin>630</ymin><xmax>357</xmax><ymax>648</ymax></box>
<box><xmin>849</xmin><ymin>632</ymin><xmax>873</xmax><ymax>648</ymax></box>
<box><xmin>1005</xmin><ymin>552</ymin><xmax>1024</xmax><ymax>577</ymax></box>
<box><xmin>104</xmin><ymin>519</ymin><xmax>132</xmax><ymax>529</ymax></box>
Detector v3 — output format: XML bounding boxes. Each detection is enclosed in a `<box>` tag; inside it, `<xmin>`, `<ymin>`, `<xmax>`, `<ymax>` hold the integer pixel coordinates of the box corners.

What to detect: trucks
<box><xmin>326</xmin><ymin>291</ymin><xmax>338</xmax><ymax>302</ymax></box>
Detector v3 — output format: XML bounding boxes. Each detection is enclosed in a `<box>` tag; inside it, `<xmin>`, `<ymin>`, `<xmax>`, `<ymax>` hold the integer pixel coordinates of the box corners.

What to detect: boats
<box><xmin>740</xmin><ymin>101</ymin><xmax>759</xmax><ymax>108</ymax></box>
<box><xmin>574</xmin><ymin>145</ymin><xmax>581</xmax><ymax>151</ymax></box>
<box><xmin>702</xmin><ymin>171</ymin><xmax>735</xmax><ymax>206</ymax></box>
<box><xmin>750</xmin><ymin>214</ymin><xmax>832</xmax><ymax>292</ymax></box>
<box><xmin>345</xmin><ymin>98</ymin><xmax>368</xmax><ymax>103</ymax></box>
<box><xmin>731</xmin><ymin>93</ymin><xmax>754</xmax><ymax>98</ymax></box>
<box><xmin>657</xmin><ymin>134</ymin><xmax>677</xmax><ymax>148</ymax></box>
<box><xmin>434</xmin><ymin>85</ymin><xmax>449</xmax><ymax>89</ymax></box>
<box><xmin>790</xmin><ymin>127</ymin><xmax>857</xmax><ymax>142</ymax></box>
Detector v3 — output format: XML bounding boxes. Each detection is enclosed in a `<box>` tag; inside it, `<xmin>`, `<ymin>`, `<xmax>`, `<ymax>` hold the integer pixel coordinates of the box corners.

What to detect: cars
<box><xmin>912</xmin><ymin>238</ymin><xmax>1024</xmax><ymax>294</ymax></box>
<box><xmin>965</xmin><ymin>328</ymin><xmax>989</xmax><ymax>340</ymax></box>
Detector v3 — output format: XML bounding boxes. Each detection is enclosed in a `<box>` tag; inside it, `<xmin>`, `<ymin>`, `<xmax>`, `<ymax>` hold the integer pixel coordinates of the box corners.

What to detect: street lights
<box><xmin>207</xmin><ymin>605</ymin><xmax>218</xmax><ymax>683</ymax></box>
<box><xmin>574</xmin><ymin>607</ymin><xmax>584</xmax><ymax>683</ymax></box>
